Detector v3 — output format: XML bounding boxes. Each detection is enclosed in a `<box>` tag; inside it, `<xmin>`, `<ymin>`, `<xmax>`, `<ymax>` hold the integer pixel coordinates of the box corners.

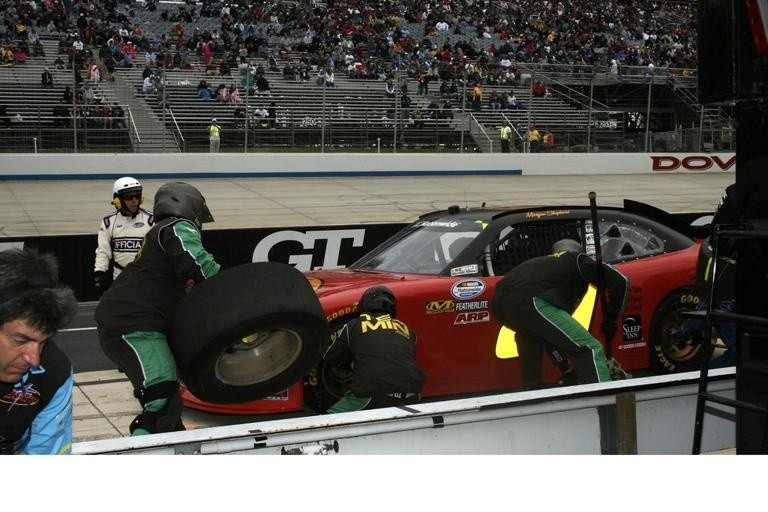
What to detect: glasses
<box><xmin>122</xmin><ymin>193</ymin><xmax>140</xmax><ymax>201</ymax></box>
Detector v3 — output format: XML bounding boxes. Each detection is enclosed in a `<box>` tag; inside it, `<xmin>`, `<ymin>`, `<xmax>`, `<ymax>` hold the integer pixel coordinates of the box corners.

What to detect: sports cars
<box><xmin>180</xmin><ymin>207</ymin><xmax>722</xmax><ymax>415</ymax></box>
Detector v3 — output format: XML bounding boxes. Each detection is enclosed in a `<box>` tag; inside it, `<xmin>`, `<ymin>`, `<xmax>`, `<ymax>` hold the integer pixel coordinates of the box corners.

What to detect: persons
<box><xmin>501</xmin><ymin>121</ymin><xmax>512</xmax><ymax>153</ymax></box>
<box><xmin>492</xmin><ymin>239</ymin><xmax>629</xmax><ymax>390</ymax></box>
<box><xmin>527</xmin><ymin>126</ymin><xmax>553</xmax><ymax>153</ymax></box>
<box><xmin>323</xmin><ymin>286</ymin><xmax>425</xmax><ymax>413</ymax></box>
<box><xmin>92</xmin><ymin>176</ymin><xmax>155</xmax><ymax>286</ymax></box>
<box><xmin>207</xmin><ymin>119</ymin><xmax>221</xmax><ymax>153</ymax></box>
<box><xmin>682</xmin><ymin>181</ymin><xmax>738</xmax><ymax>368</ymax></box>
<box><xmin>1</xmin><ymin>0</ymin><xmax>700</xmax><ymax>136</ymax></box>
<box><xmin>95</xmin><ymin>182</ymin><xmax>222</xmax><ymax>438</ymax></box>
<box><xmin>722</xmin><ymin>123</ymin><xmax>733</xmax><ymax>149</ymax></box>
<box><xmin>1</xmin><ymin>247</ymin><xmax>79</xmax><ymax>455</ymax></box>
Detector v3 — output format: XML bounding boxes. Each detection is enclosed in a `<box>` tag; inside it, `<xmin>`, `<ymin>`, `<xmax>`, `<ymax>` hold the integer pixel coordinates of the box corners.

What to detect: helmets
<box><xmin>153</xmin><ymin>182</ymin><xmax>214</xmax><ymax>230</ymax></box>
<box><xmin>111</xmin><ymin>177</ymin><xmax>144</xmax><ymax>216</ymax></box>
<box><xmin>358</xmin><ymin>285</ymin><xmax>395</xmax><ymax>318</ymax></box>
<box><xmin>554</xmin><ymin>239</ymin><xmax>582</xmax><ymax>253</ymax></box>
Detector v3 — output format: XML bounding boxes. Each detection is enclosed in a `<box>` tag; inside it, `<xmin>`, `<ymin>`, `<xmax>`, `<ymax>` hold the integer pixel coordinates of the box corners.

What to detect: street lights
<box><xmin>643</xmin><ymin>61</ymin><xmax>655</xmax><ymax>150</ymax></box>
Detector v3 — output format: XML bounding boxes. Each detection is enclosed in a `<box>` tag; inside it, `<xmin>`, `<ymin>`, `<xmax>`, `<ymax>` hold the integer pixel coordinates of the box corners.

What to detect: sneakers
<box><xmin>707</xmin><ymin>349</ymin><xmax>738</xmax><ymax>368</ymax></box>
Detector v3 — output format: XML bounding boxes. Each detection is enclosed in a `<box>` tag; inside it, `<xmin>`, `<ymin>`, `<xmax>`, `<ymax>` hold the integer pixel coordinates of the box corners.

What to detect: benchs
<box><xmin>0</xmin><ymin>20</ymin><xmax>132</xmax><ymax>149</ymax></box>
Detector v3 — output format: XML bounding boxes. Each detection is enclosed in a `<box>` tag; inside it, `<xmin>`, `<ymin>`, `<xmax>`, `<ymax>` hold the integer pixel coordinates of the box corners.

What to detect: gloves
<box><xmin>563</xmin><ymin>368</ymin><xmax>579</xmax><ymax>385</ymax></box>
<box><xmin>600</xmin><ymin>321</ymin><xmax>617</xmax><ymax>340</ymax></box>
<box><xmin>91</xmin><ymin>271</ymin><xmax>108</xmax><ymax>299</ymax></box>
